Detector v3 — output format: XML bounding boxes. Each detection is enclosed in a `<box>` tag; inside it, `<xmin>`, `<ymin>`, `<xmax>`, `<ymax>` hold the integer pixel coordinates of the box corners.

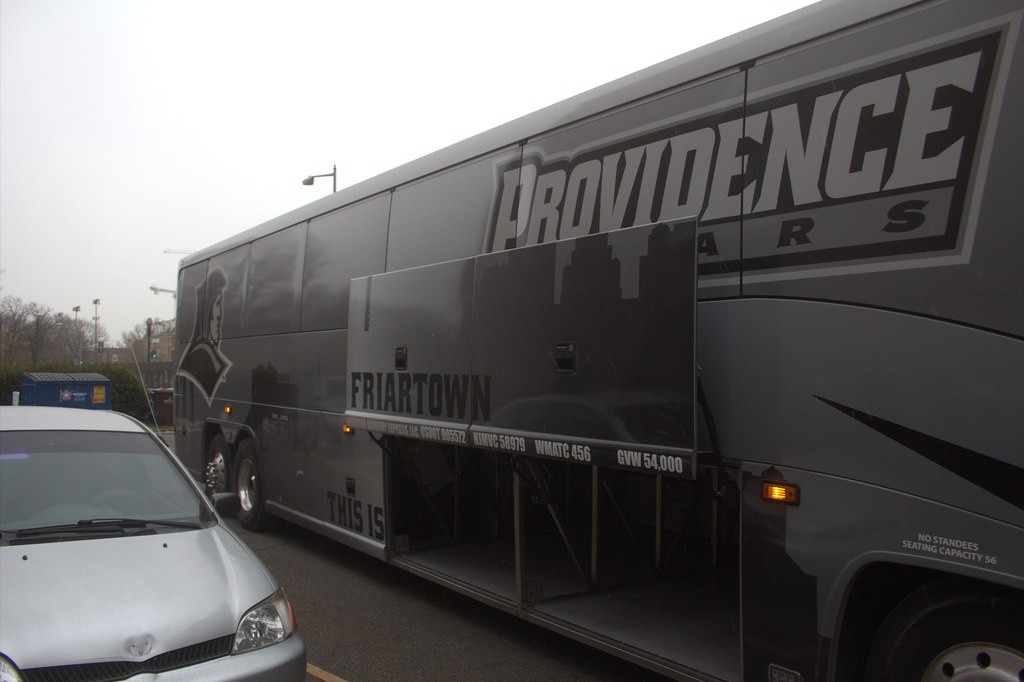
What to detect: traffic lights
<box><xmin>150</xmin><ymin>349</ymin><xmax>157</xmax><ymax>359</ymax></box>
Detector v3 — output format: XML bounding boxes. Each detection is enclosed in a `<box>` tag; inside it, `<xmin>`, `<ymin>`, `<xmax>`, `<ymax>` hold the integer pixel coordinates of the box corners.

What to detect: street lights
<box><xmin>145</xmin><ymin>317</ymin><xmax>154</xmax><ymax>385</ymax></box>
<box><xmin>301</xmin><ymin>163</ymin><xmax>336</xmax><ymax>193</ymax></box>
<box><xmin>73</xmin><ymin>306</ymin><xmax>81</xmax><ymax>330</ymax></box>
<box><xmin>93</xmin><ymin>298</ymin><xmax>101</xmax><ymax>366</ymax></box>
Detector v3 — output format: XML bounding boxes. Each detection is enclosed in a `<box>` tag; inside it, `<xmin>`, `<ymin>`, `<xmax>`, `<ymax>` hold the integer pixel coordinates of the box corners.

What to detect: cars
<box><xmin>0</xmin><ymin>401</ymin><xmax>307</xmax><ymax>682</ymax></box>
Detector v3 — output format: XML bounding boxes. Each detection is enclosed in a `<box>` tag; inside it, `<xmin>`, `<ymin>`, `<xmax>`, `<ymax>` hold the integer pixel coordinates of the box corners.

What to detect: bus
<box><xmin>167</xmin><ymin>0</ymin><xmax>1024</xmax><ymax>682</ymax></box>
